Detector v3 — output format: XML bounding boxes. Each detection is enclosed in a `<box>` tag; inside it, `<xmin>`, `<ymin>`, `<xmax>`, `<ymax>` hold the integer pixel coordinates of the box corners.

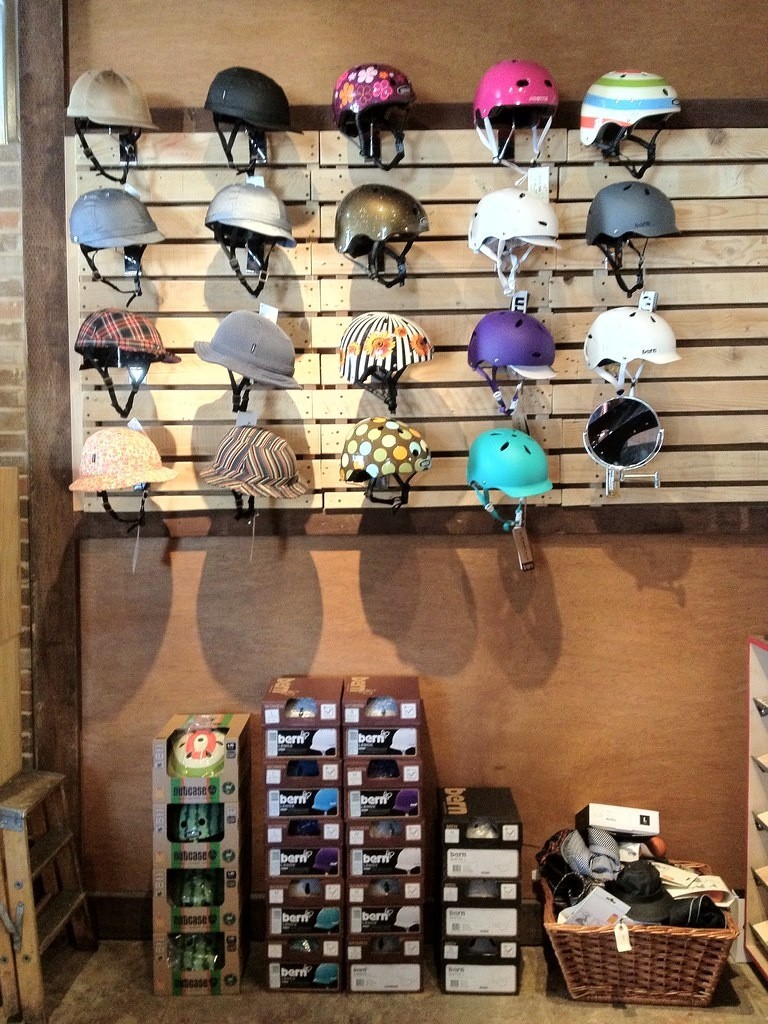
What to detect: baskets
<box><xmin>540</xmin><ymin>858</ymin><xmax>740</xmax><ymax>1008</ymax></box>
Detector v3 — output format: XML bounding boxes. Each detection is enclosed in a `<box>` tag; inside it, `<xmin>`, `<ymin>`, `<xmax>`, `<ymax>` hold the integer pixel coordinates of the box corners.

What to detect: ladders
<box><xmin>1</xmin><ymin>768</ymin><xmax>99</xmax><ymax>1023</ymax></box>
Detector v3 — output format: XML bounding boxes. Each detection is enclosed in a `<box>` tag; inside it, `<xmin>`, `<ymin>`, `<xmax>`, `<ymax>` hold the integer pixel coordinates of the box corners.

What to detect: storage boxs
<box><xmin>151</xmin><ymin>674</ymin><xmax>519</xmax><ymax>994</ymax></box>
<box><xmin>574</xmin><ymin>803</ymin><xmax>660</xmax><ymax>841</ymax></box>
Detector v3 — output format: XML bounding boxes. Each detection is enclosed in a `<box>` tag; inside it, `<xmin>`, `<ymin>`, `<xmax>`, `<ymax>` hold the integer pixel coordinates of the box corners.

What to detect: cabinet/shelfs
<box><xmin>745</xmin><ymin>635</ymin><xmax>768</xmax><ymax>990</ymax></box>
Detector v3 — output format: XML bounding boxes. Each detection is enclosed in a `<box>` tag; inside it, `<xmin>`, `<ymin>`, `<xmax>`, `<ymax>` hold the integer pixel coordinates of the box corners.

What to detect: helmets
<box><xmin>579</xmin><ymin>70</ymin><xmax>683</xmax><ymax>147</ymax></box>
<box><xmin>584</xmin><ymin>306</ymin><xmax>682</xmax><ymax>369</ymax></box>
<box><xmin>586</xmin><ymin>180</ymin><xmax>682</xmax><ymax>246</ymax></box>
<box><xmin>465</xmin><ymin>427</ymin><xmax>554</xmax><ymax>499</ymax></box>
<box><xmin>330</xmin><ymin>62</ymin><xmax>417</xmax><ymax>138</ymax></box>
<box><xmin>337</xmin><ymin>312</ymin><xmax>434</xmax><ymax>385</ymax></box>
<box><xmin>467</xmin><ymin>310</ymin><xmax>556</xmax><ymax>370</ymax></box>
<box><xmin>467</xmin><ymin>187</ymin><xmax>560</xmax><ymax>252</ymax></box>
<box><xmin>337</xmin><ymin>416</ymin><xmax>433</xmax><ymax>484</ymax></box>
<box><xmin>334</xmin><ymin>184</ymin><xmax>430</xmax><ymax>259</ymax></box>
<box><xmin>472</xmin><ymin>59</ymin><xmax>558</xmax><ymax>128</ymax></box>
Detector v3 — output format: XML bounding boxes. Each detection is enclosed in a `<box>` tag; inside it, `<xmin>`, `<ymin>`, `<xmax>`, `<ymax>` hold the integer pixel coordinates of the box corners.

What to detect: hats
<box><xmin>74</xmin><ymin>308</ymin><xmax>182</xmax><ymax>365</ymax></box>
<box><xmin>66</xmin><ymin>66</ymin><xmax>160</xmax><ymax>131</ymax></box>
<box><xmin>69</xmin><ymin>188</ymin><xmax>167</xmax><ymax>248</ymax></box>
<box><xmin>205</xmin><ymin>183</ymin><xmax>298</xmax><ymax>249</ymax></box>
<box><xmin>193</xmin><ymin>309</ymin><xmax>303</xmax><ymax>392</ymax></box>
<box><xmin>204</xmin><ymin>66</ymin><xmax>304</xmax><ymax>135</ymax></box>
<box><xmin>199</xmin><ymin>425</ymin><xmax>310</xmax><ymax>500</ymax></box>
<box><xmin>69</xmin><ymin>426</ymin><xmax>177</xmax><ymax>493</ymax></box>
